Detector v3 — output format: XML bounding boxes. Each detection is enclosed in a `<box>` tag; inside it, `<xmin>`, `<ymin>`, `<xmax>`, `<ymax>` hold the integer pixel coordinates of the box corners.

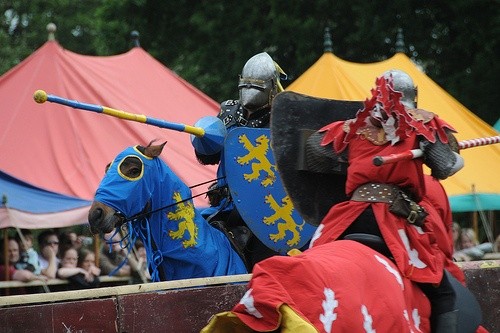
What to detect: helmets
<box><xmin>239</xmin><ymin>52</ymin><xmax>278</xmax><ymax>110</ymax></box>
<box><xmin>379</xmin><ymin>68</ymin><xmax>418</xmax><ymax>109</ymax></box>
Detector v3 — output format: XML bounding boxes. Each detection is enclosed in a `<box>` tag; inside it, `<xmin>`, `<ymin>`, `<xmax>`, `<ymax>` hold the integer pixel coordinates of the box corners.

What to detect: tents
<box><xmin>285</xmin><ymin>28</ymin><xmax>500</xmax><ymax>211</ymax></box>
<box><xmin>0</xmin><ymin>23</ymin><xmax>222</xmax><ymax>230</ymax></box>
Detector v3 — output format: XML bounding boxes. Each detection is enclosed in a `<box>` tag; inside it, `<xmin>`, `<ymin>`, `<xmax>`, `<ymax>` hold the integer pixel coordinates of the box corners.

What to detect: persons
<box><xmin>308</xmin><ymin>69</ymin><xmax>464</xmax><ymax>333</ymax></box>
<box><xmin>1</xmin><ymin>218</ymin><xmax>155</xmax><ymax>291</ymax></box>
<box><xmin>193</xmin><ymin>51</ymin><xmax>315</xmax><ymax>272</ymax></box>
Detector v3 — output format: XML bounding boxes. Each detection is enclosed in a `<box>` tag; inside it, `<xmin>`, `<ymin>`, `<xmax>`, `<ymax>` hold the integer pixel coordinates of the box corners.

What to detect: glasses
<box><xmin>47</xmin><ymin>241</ymin><xmax>59</xmax><ymax>245</ymax></box>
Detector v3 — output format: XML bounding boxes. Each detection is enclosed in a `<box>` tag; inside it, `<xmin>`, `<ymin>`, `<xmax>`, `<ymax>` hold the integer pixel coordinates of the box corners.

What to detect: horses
<box><xmin>87</xmin><ymin>140</ymin><xmax>249</xmax><ymax>283</ymax></box>
<box><xmin>200</xmin><ymin>179</ymin><xmax>457</xmax><ymax>333</ymax></box>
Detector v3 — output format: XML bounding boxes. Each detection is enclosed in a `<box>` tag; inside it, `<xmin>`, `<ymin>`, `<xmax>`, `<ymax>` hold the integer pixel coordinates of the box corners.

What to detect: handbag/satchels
<box><xmin>388</xmin><ymin>191</ymin><xmax>430</xmax><ymax>227</ymax></box>
